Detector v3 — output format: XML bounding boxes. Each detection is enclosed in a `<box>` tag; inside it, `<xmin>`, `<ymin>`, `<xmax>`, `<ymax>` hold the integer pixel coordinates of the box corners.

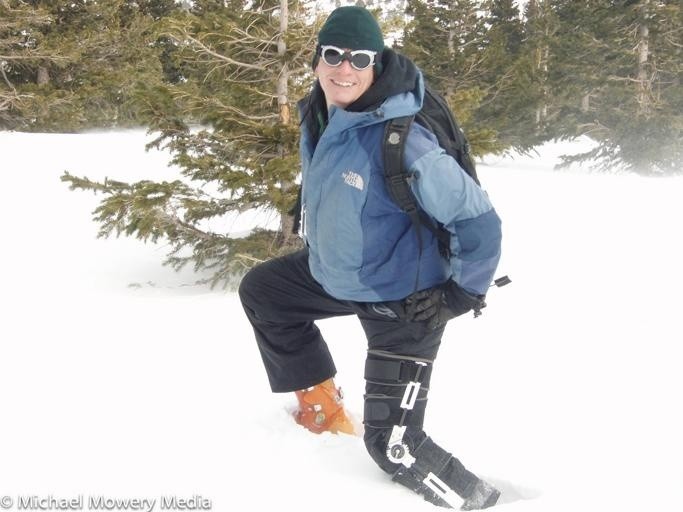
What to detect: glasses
<box><xmin>321</xmin><ymin>45</ymin><xmax>378</xmax><ymax>71</ymax></box>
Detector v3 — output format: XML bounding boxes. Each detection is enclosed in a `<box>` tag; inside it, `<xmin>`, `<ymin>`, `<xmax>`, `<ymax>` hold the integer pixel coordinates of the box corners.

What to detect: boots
<box><xmin>295</xmin><ymin>377</ymin><xmax>354</xmax><ymax>435</ymax></box>
<box><xmin>392</xmin><ymin>437</ymin><xmax>500</xmax><ymax>510</ymax></box>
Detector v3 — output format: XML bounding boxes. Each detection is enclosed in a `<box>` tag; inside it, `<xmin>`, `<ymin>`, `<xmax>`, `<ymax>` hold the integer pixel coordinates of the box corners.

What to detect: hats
<box><xmin>312</xmin><ymin>6</ymin><xmax>384</xmax><ymax>83</ymax></box>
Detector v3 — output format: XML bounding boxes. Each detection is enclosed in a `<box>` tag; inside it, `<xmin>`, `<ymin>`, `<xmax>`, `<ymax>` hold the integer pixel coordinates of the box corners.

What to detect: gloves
<box><xmin>406</xmin><ymin>275</ymin><xmax>486</xmax><ymax>330</ymax></box>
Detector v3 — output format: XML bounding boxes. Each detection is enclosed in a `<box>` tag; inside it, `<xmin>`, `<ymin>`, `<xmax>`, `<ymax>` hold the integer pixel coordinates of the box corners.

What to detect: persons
<box><xmin>237</xmin><ymin>5</ymin><xmax>502</xmax><ymax>509</ymax></box>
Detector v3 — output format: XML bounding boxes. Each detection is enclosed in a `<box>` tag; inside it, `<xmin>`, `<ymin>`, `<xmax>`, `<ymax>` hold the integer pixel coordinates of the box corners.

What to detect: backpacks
<box><xmin>292</xmin><ymin>86</ymin><xmax>481</xmax><ymax>261</ymax></box>
